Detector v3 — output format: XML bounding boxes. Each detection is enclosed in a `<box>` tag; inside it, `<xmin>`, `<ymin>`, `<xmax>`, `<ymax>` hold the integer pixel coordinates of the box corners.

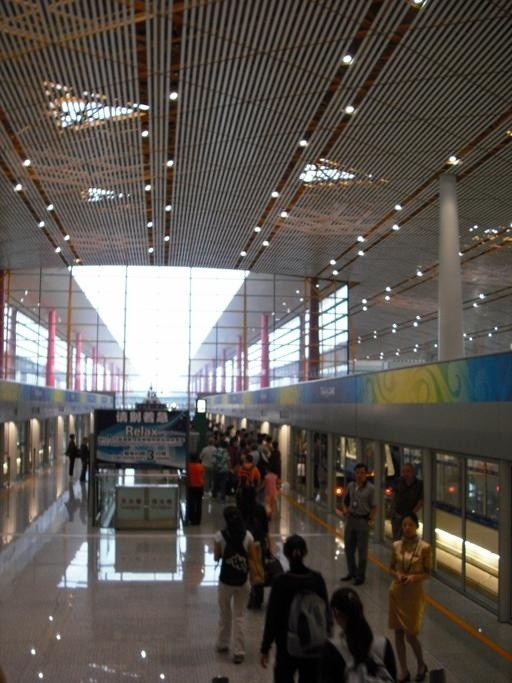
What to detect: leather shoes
<box><xmin>353</xmin><ymin>580</ymin><xmax>364</xmax><ymax>585</ymax></box>
<box><xmin>341</xmin><ymin>575</ymin><xmax>351</xmax><ymax>581</ymax></box>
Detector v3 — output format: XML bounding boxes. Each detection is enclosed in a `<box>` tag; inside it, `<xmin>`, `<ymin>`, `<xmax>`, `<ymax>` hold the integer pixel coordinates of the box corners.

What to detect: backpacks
<box><xmin>328</xmin><ymin>636</ymin><xmax>396</xmax><ymax>683</ymax></box>
<box><xmin>235</xmin><ymin>464</ymin><xmax>255</xmax><ymax>499</ymax></box>
<box><xmin>283</xmin><ymin>573</ymin><xmax>329</xmax><ymax>659</ymax></box>
<box><xmin>214</xmin><ymin>450</ymin><xmax>228</xmax><ymax>475</ymax></box>
<box><xmin>219</xmin><ymin>530</ymin><xmax>249</xmax><ymax>586</ymax></box>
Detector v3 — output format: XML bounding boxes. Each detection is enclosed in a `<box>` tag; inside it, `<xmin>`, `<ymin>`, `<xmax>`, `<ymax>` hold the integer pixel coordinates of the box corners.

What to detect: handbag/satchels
<box><xmin>258</xmin><ymin>452</ymin><xmax>268</xmax><ymax>467</ymax></box>
<box><xmin>246</xmin><ymin>540</ymin><xmax>265</xmax><ymax>588</ymax></box>
<box><xmin>263</xmin><ymin>548</ymin><xmax>285</xmax><ymax>583</ymax></box>
<box><xmin>344</xmin><ymin>487</ymin><xmax>350</xmax><ymax>506</ymax></box>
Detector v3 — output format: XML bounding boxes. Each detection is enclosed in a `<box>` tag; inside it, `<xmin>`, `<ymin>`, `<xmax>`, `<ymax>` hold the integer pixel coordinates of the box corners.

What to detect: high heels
<box><xmin>395</xmin><ymin>670</ymin><xmax>410</xmax><ymax>683</ymax></box>
<box><xmin>415</xmin><ymin>663</ymin><xmax>428</xmax><ymax>682</ymax></box>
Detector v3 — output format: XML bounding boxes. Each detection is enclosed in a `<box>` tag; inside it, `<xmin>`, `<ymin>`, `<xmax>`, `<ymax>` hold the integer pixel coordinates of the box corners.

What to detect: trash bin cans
<box><xmin>114</xmin><ymin>484</ymin><xmax>179</xmax><ymax>530</ymax></box>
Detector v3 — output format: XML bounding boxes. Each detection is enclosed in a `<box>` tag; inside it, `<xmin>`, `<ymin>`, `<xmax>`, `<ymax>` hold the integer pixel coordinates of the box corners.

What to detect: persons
<box><xmin>177</xmin><ymin>410</ymin><xmax>284</xmax><ymax>533</ymax></box>
<box><xmin>66</xmin><ymin>433</ymin><xmax>77</xmax><ymax>475</ymax></box>
<box><xmin>182</xmin><ymin>536</ymin><xmax>206</xmax><ymax>593</ymax></box>
<box><xmin>232</xmin><ymin>482</ymin><xmax>266</xmax><ymax>610</ymax></box>
<box><xmin>386</xmin><ymin>511</ymin><xmax>435</xmax><ymax>683</ymax></box>
<box><xmin>339</xmin><ymin>463</ymin><xmax>378</xmax><ymax>586</ymax></box>
<box><xmin>78</xmin><ymin>480</ymin><xmax>87</xmax><ymax>525</ymax></box>
<box><xmin>387</xmin><ymin>462</ymin><xmax>423</xmax><ymax>537</ymax></box>
<box><xmin>64</xmin><ymin>479</ymin><xmax>76</xmax><ymax>522</ymax></box>
<box><xmin>79</xmin><ymin>437</ymin><xmax>89</xmax><ymax>482</ymax></box>
<box><xmin>212</xmin><ymin>502</ymin><xmax>263</xmax><ymax>664</ymax></box>
<box><xmin>296</xmin><ymin>637</ymin><xmax>348</xmax><ymax>683</ymax></box>
<box><xmin>257</xmin><ymin>534</ymin><xmax>333</xmax><ymax>682</ymax></box>
<box><xmin>328</xmin><ymin>586</ymin><xmax>397</xmax><ymax>682</ymax></box>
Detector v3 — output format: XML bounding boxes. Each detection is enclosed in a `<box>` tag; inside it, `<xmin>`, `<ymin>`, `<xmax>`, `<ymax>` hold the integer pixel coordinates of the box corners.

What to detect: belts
<box><xmin>396</xmin><ymin>510</ymin><xmax>404</xmax><ymax>515</ymax></box>
<box><xmin>350</xmin><ymin>513</ymin><xmax>369</xmax><ymax>518</ymax></box>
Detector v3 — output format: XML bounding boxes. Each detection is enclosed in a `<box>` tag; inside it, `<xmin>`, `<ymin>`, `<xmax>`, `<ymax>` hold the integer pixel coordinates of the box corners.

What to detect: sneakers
<box><xmin>233</xmin><ymin>654</ymin><xmax>244</xmax><ymax>664</ymax></box>
<box><xmin>220</xmin><ymin>495</ymin><xmax>228</xmax><ymax>504</ymax></box>
<box><xmin>215</xmin><ymin>646</ymin><xmax>229</xmax><ymax>657</ymax></box>
